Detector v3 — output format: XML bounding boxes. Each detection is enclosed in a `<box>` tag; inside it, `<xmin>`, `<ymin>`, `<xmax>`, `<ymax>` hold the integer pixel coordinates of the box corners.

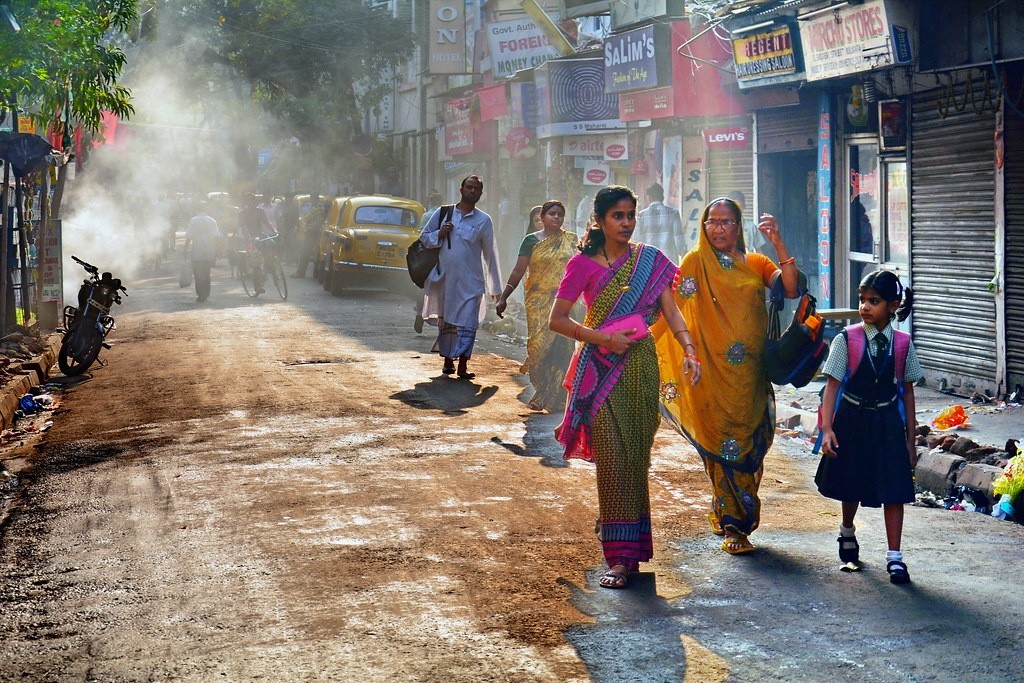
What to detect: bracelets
<box><xmin>778</xmin><ymin>257</ymin><xmax>795</xmax><ymax>265</ymax></box>
<box><xmin>506</xmin><ymin>284</ymin><xmax>515</xmax><ymax>290</ymax></box>
<box><xmin>575</xmin><ymin>324</ymin><xmax>583</xmax><ymax>341</ymax></box>
<box><xmin>674</xmin><ymin>330</ymin><xmax>700</xmax><ymax>361</ymax></box>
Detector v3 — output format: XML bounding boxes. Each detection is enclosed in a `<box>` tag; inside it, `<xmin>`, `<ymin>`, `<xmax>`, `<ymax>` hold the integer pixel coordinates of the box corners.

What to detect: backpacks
<box><xmin>764</xmin><ymin>267</ymin><xmax>827</xmax><ymax>387</ymax></box>
<box><xmin>819</xmin><ymin>324</ymin><xmax>911</xmax><ymax>431</ymax></box>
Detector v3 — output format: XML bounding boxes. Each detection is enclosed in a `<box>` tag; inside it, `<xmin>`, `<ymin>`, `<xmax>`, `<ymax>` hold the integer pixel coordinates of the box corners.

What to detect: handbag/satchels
<box><xmin>599</xmin><ymin>314</ymin><xmax>647</xmax><ymax>355</ymax></box>
<box><xmin>406</xmin><ymin>205</ymin><xmax>454</xmax><ymax>289</ymax></box>
<box><xmin>180</xmin><ymin>258</ymin><xmax>193</xmax><ymax>288</ymax></box>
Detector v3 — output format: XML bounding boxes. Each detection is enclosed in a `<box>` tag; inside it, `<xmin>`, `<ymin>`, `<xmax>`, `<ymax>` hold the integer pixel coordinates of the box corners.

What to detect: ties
<box><xmin>873</xmin><ymin>333</ymin><xmax>888</xmax><ymax>358</ymax></box>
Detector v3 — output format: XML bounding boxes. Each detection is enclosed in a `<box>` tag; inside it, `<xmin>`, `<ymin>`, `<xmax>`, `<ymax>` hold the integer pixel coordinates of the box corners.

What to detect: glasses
<box><xmin>704</xmin><ymin>220</ymin><xmax>739</xmax><ymax>230</ymax></box>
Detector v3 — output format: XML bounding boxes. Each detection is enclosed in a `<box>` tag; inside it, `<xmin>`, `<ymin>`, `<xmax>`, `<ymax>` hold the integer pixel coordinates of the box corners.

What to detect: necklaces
<box><xmin>602</xmin><ymin>243</ymin><xmax>633</xmax><ymax>292</ymax></box>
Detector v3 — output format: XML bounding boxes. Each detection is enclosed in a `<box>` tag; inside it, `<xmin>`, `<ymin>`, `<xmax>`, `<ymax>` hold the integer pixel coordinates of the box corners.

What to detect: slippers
<box><xmin>708</xmin><ymin>511</ymin><xmax>725</xmax><ymax>534</ymax></box>
<box><xmin>722</xmin><ymin>537</ymin><xmax>756</xmax><ymax>553</ymax></box>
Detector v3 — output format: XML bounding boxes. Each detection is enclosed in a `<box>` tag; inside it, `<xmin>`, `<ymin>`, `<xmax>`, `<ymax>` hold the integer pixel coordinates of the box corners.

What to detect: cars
<box><xmin>207</xmin><ymin>192</ymin><xmax>230</xmax><ymax>206</ymax></box>
<box><xmin>329</xmin><ymin>193</ymin><xmax>427</xmax><ymax>296</ymax></box>
<box><xmin>254</xmin><ymin>194</ymin><xmax>370</xmax><ymax>285</ymax></box>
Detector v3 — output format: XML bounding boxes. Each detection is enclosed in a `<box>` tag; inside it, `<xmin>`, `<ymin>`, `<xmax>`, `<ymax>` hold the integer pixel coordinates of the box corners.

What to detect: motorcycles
<box><xmin>56</xmin><ymin>255</ymin><xmax>128</xmax><ymax>377</ymax></box>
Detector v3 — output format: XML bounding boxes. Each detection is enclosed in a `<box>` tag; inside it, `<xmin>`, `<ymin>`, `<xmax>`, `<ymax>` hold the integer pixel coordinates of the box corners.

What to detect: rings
<box><xmin>446</xmin><ymin>224</ymin><xmax>449</xmax><ymax>229</ymax></box>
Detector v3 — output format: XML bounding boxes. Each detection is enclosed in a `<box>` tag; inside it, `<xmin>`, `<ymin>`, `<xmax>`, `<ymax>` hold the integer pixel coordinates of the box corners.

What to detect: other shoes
<box><xmin>443</xmin><ymin>364</ymin><xmax>456</xmax><ymax>373</ymax></box>
<box><xmin>255</xmin><ymin>287</ymin><xmax>265</xmax><ymax>293</ymax></box>
<box><xmin>837</xmin><ymin>532</ymin><xmax>859</xmax><ymax>562</ymax></box>
<box><xmin>414</xmin><ymin>314</ymin><xmax>424</xmax><ymax>333</ymax></box>
<box><xmin>887</xmin><ymin>561</ymin><xmax>909</xmax><ymax>583</ymax></box>
<box><xmin>457</xmin><ymin>369</ymin><xmax>475</xmax><ymax>378</ymax></box>
<box><xmin>289</xmin><ymin>273</ymin><xmax>305</xmax><ymax>278</ymax></box>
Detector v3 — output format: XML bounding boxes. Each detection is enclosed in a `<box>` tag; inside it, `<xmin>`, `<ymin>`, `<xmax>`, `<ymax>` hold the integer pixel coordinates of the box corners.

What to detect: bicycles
<box><xmin>235</xmin><ymin>233</ymin><xmax>289</xmax><ymax>300</ymax></box>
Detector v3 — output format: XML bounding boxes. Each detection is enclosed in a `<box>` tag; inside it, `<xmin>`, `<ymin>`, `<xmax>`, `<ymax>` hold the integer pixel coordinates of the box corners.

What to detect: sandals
<box><xmin>599</xmin><ymin>565</ymin><xmax>626</xmax><ymax>587</ymax></box>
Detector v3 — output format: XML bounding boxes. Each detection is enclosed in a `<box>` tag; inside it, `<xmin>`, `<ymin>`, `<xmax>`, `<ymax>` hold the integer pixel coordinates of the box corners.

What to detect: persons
<box><xmin>496</xmin><ymin>200</ymin><xmax>578</xmax><ymax>413</ymax></box>
<box><xmin>548</xmin><ymin>185</ymin><xmax>701</xmax><ymax>587</ymax></box>
<box><xmin>815</xmin><ymin>271</ymin><xmax>923</xmax><ymax>584</ymax></box>
<box><xmin>182</xmin><ymin>183</ymin><xmax>772</xmax><ymax>333</ymax></box>
<box><xmin>651</xmin><ymin>196</ymin><xmax>799</xmax><ymax>555</ymax></box>
<box><xmin>421</xmin><ymin>175</ymin><xmax>503</xmax><ymax>377</ymax></box>
